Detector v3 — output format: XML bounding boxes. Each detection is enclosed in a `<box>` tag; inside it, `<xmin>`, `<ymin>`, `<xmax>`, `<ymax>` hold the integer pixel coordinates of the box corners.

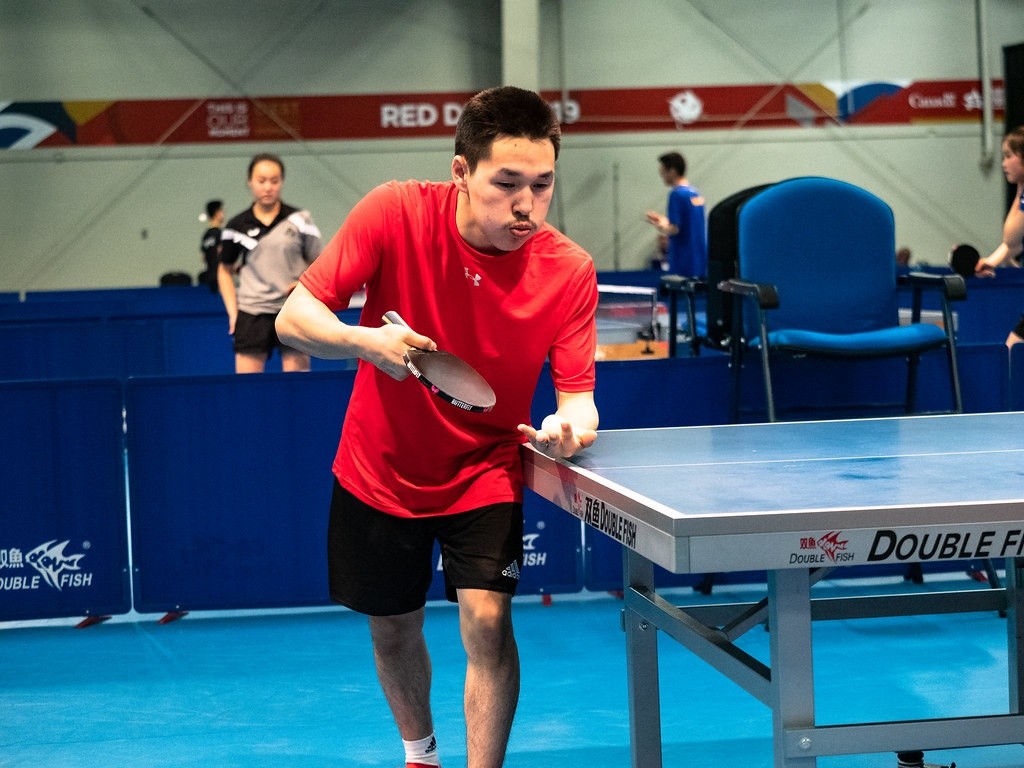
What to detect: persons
<box><xmin>214</xmin><ymin>153</ymin><xmax>324</xmax><ymax>373</ymax></box>
<box><xmin>273</xmin><ymin>86</ymin><xmax>602</xmax><ymax>768</ymax></box>
<box><xmin>198</xmin><ymin>203</ymin><xmax>238</xmax><ymax>286</ymax></box>
<box><xmin>644</xmin><ymin>150</ymin><xmax>709</xmax><ymax>279</ymax></box>
<box><xmin>973</xmin><ymin>124</ymin><xmax>1024</xmax><ymax>347</ymax></box>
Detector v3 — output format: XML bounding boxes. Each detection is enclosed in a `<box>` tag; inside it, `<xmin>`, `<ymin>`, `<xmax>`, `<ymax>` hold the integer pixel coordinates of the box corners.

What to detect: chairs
<box><xmin>705</xmin><ymin>175</ymin><xmax>1010</xmax><ymax>620</ymax></box>
<box><xmin>657</xmin><ymin>179</ymin><xmax>787</xmax><ymax>356</ymax></box>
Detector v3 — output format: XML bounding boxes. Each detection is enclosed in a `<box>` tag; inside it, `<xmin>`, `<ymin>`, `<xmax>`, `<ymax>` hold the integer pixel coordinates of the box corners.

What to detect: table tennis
<box><xmin>542</xmin><ymin>414</ymin><xmax>565</xmax><ymax>436</ymax></box>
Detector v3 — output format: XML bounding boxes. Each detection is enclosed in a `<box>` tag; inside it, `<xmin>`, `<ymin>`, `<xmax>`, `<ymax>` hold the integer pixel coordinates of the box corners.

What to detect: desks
<box><xmin>514</xmin><ymin>410</ymin><xmax>1024</xmax><ymax>768</ymax></box>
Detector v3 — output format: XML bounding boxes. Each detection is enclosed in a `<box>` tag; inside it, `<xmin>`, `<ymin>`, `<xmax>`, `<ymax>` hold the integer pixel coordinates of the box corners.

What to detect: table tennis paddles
<box><xmin>951</xmin><ymin>242</ymin><xmax>996</xmax><ymax>277</ymax></box>
<box><xmin>381</xmin><ymin>309</ymin><xmax>497</xmax><ymax>414</ymax></box>
